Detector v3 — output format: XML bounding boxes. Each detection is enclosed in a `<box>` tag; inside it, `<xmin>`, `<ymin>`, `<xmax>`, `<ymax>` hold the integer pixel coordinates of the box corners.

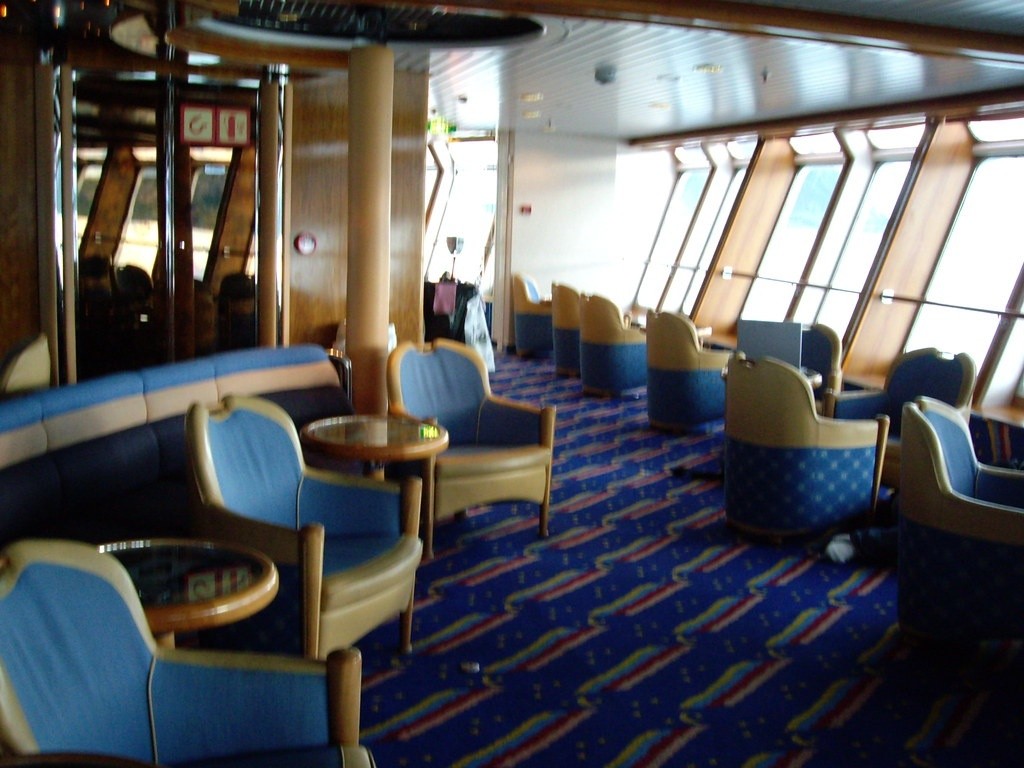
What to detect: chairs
<box><xmin>510</xmin><ymin>272</ymin><xmax>1024</xmax><ymax>657</ymax></box>
<box><xmin>0</xmin><ymin>532</ymin><xmax>374</xmax><ymax>767</ymax></box>
<box><xmin>183</xmin><ymin>393</ymin><xmax>427</xmax><ymax>658</ymax></box>
<box><xmin>384</xmin><ymin>337</ymin><xmax>557</xmax><ymax>562</ymax></box>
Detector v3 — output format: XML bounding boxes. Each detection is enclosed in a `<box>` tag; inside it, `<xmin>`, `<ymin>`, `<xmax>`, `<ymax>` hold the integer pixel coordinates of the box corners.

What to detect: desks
<box><xmin>301</xmin><ymin>413</ymin><xmax>451</xmax><ymax>481</ymax></box>
<box><xmin>81</xmin><ymin>535</ymin><xmax>282</xmax><ymax>656</ymax></box>
